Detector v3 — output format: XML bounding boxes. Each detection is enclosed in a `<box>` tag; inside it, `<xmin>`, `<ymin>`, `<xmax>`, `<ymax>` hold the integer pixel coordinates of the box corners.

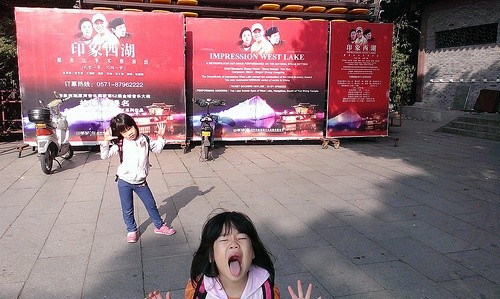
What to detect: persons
<box><xmin>75</xmin><ymin>13</ymin><xmax>136</xmax><ymax>48</ymax></box>
<box><xmin>240</xmin><ymin>23</ymin><xmax>292</xmax><ymax>54</ymax></box>
<box><xmin>144</xmin><ymin>207</ymin><xmax>322</xmax><ymax>299</ymax></box>
<box><xmin>348</xmin><ymin>26</ymin><xmax>375</xmax><ymax>44</ymax></box>
<box><xmin>100</xmin><ymin>113</ymin><xmax>176</xmax><ymax>243</ymax></box>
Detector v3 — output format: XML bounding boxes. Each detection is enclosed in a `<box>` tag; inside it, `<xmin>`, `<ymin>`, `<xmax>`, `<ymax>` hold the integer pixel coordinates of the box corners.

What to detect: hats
<box><xmin>265</xmin><ymin>26</ymin><xmax>280</xmax><ymax>36</ymax></box>
<box><xmin>250</xmin><ymin>22</ymin><xmax>263</xmax><ymax>33</ymax></box>
<box><xmin>356</xmin><ymin>27</ymin><xmax>363</xmax><ymax>32</ymax></box>
<box><xmin>92</xmin><ymin>13</ymin><xmax>106</xmax><ymax>23</ymax></box>
<box><xmin>109</xmin><ymin>17</ymin><xmax>125</xmax><ymax>28</ymax></box>
<box><xmin>364</xmin><ymin>29</ymin><xmax>371</xmax><ymax>34</ymax></box>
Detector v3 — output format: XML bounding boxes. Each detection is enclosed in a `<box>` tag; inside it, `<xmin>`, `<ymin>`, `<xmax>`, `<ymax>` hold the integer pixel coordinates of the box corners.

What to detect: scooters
<box><xmin>27</xmin><ymin>90</ymin><xmax>74</xmax><ymax>174</ymax></box>
<box><xmin>190</xmin><ymin>97</ymin><xmax>226</xmax><ymax>163</ymax></box>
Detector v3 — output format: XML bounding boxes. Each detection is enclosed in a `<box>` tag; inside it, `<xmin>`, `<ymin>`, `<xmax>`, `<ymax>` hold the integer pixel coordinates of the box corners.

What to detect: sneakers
<box><xmin>154</xmin><ymin>223</ymin><xmax>176</xmax><ymax>236</ymax></box>
<box><xmin>126</xmin><ymin>231</ymin><xmax>138</xmax><ymax>243</ymax></box>
